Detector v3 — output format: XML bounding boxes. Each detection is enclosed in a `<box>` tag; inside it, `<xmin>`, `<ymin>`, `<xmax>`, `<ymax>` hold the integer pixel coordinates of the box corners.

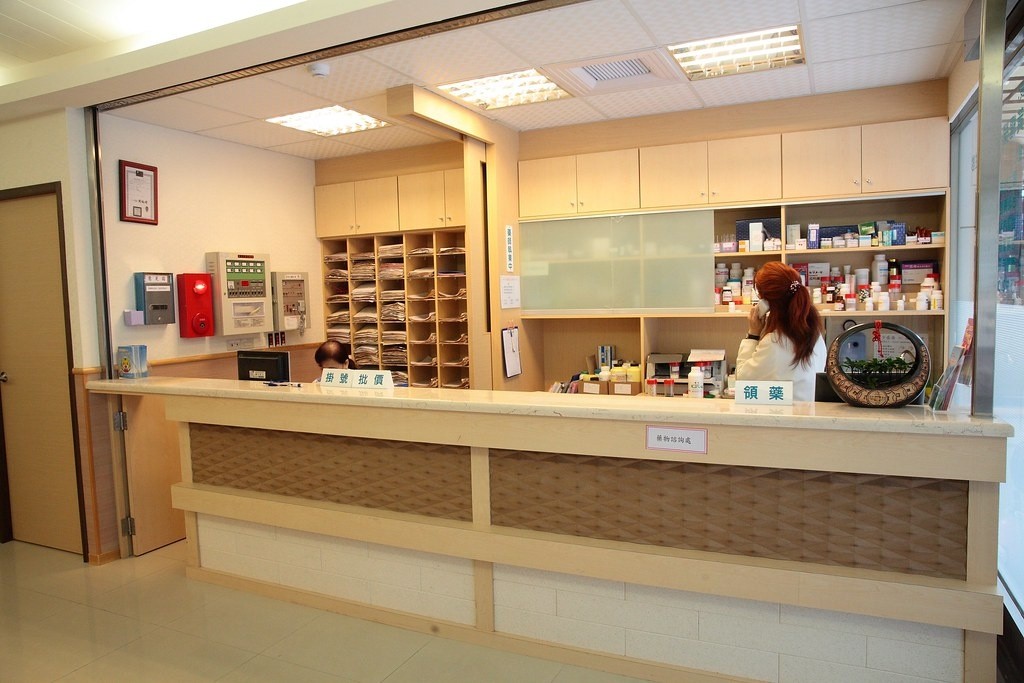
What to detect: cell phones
<box><xmin>756</xmin><ymin>299</ymin><xmax>770</xmax><ymax>320</ymax></box>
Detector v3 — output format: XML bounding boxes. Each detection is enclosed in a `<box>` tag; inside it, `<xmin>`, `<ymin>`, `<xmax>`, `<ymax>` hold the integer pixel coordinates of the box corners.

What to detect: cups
<box><xmin>664</xmin><ymin>380</ymin><xmax>674</xmax><ymax>397</ymax></box>
<box><xmin>669</xmin><ymin>362</ymin><xmax>679</xmax><ymax>380</ymax></box>
<box><xmin>853</xmin><ymin>268</ymin><xmax>870</xmax><ymax>290</ymax></box>
<box><xmin>647</xmin><ymin>379</ymin><xmax>657</xmax><ymax>396</ymax></box>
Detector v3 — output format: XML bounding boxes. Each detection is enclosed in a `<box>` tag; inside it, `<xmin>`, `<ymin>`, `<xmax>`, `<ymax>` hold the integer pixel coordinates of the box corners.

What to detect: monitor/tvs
<box><xmin>237</xmin><ymin>351</ymin><xmax>290</xmax><ymax>382</ymax></box>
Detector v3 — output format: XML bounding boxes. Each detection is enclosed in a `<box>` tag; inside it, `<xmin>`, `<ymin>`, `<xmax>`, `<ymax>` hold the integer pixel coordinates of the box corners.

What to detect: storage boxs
<box><xmin>579</xmin><ymin>348</ymin><xmax>728</xmax><ymax>399</ymax></box>
<box><xmin>714</xmin><ymin>216</ymin><xmax>944</xmax><ymax>286</ymax></box>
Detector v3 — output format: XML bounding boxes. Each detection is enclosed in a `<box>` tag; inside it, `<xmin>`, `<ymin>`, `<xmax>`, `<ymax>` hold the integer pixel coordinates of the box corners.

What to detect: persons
<box><xmin>312</xmin><ymin>340</ymin><xmax>357</xmax><ymax>387</ymax></box>
<box><xmin>734</xmin><ymin>260</ymin><xmax>828</xmax><ymax>405</ymax></box>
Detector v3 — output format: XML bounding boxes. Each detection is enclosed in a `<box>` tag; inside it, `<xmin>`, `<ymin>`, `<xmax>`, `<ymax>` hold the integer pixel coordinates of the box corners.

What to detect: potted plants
<box><xmin>826</xmin><ymin>320</ymin><xmax>931</xmax><ymax>407</ymax></box>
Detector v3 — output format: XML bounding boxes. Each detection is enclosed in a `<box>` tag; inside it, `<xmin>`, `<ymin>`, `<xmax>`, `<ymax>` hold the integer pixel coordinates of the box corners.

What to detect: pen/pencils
<box><xmin>268</xmin><ymin>383</ymin><xmax>300</xmax><ymax>387</ymax></box>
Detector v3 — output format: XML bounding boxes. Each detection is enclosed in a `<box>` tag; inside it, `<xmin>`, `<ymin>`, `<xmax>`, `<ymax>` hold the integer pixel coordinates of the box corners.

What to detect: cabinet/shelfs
<box><xmin>320</xmin><ymin>226</ymin><xmax>490</xmax><ymax>390</ymax></box>
<box><xmin>781</xmin><ymin>116</ymin><xmax>950</xmax><ymax>200</ymax></box>
<box><xmin>518</xmin><ymin>147</ymin><xmax>640</xmax><ymax>217</ymax></box>
<box><xmin>518</xmin><ymin>187</ymin><xmax>952</xmax><ymax>397</ymax></box>
<box><xmin>398</xmin><ymin>167</ymin><xmax>465</xmax><ymax>232</ymax></box>
<box><xmin>640</xmin><ymin>134</ymin><xmax>782</xmax><ymax>209</ymax></box>
<box><xmin>314</xmin><ymin>176</ymin><xmax>399</xmax><ymax>238</ymax></box>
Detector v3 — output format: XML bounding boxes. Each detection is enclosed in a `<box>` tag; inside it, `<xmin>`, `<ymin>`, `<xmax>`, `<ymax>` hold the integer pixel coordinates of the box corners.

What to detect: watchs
<box><xmin>743</xmin><ymin>332</ymin><xmax>761</xmax><ymax>340</ymax></box>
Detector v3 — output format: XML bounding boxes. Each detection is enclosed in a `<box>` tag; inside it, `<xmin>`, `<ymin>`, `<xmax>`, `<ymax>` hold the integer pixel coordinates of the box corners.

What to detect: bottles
<box><xmin>600</xmin><ymin>365</ymin><xmax>610</xmax><ymax>381</ymax></box>
<box><xmin>688</xmin><ymin>367</ymin><xmax>704</xmax><ymax>398</ymax></box>
<box><xmin>579</xmin><ymin>370</ymin><xmax>589</xmax><ymax>381</ymax></box>
<box><xmin>715</xmin><ymin>263</ymin><xmax>759</xmax><ymax>305</ymax></box>
<box><xmin>594</xmin><ymin>369</ymin><xmax>600</xmax><ymax>376</ymax></box>
<box><xmin>802</xmin><ymin>254</ymin><xmax>944</xmax><ymax>312</ymax></box>
